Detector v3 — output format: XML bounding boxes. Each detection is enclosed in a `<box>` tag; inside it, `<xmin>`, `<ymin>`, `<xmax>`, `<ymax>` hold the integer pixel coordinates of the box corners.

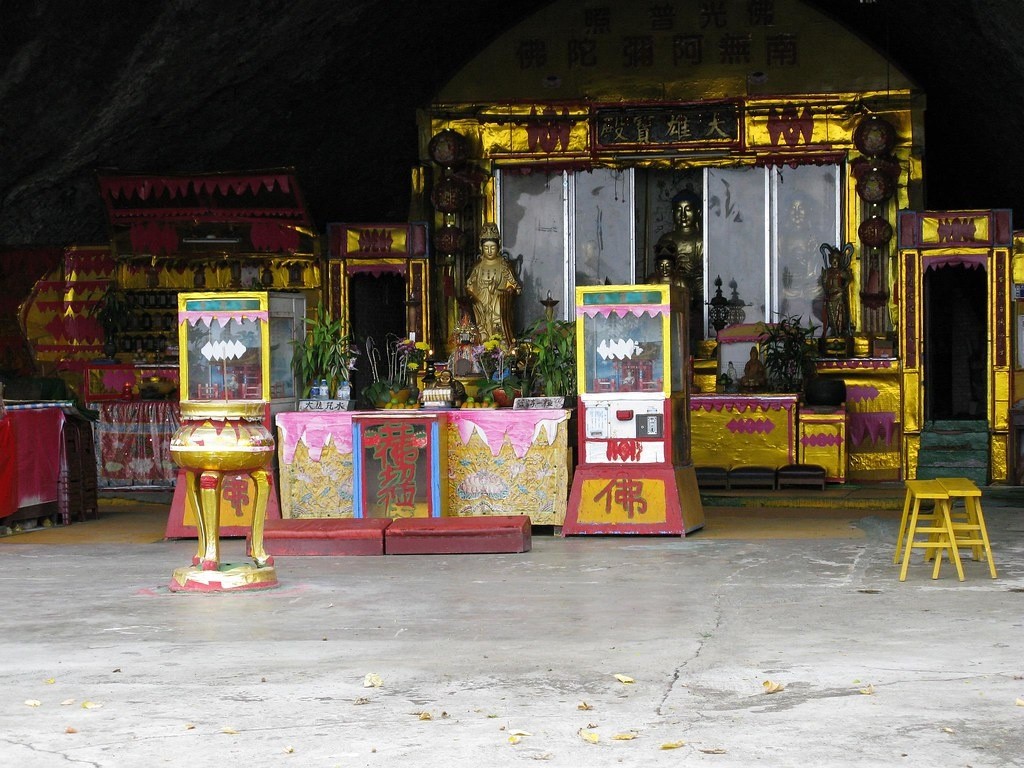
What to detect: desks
<box><xmin>275</xmin><ymin>407</ymin><xmax>576</xmax><ymax>538</ymax></box>
<box><xmin>89</xmin><ymin>402</ymin><xmax>182</xmax><ymax>492</ymax></box>
<box><xmin>687</xmin><ymin>355</ymin><xmax>907</xmax><ymax>487</ymax></box>
<box><xmin>0</xmin><ymin>406</ymin><xmax>77</xmax><ymax>529</ymax></box>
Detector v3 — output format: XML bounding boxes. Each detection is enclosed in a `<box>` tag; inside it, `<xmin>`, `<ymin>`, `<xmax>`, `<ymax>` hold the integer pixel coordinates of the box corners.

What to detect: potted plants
<box><xmin>476</xmin><ymin>374</ymin><xmax>522</xmax><ymax>406</ymax></box>
<box><xmin>361</xmin><ymin>380</ymin><xmax>411</xmax><ymax>408</ymax></box>
<box><xmin>755</xmin><ymin>311</ymin><xmax>818</xmax><ymax>393</ymax></box>
<box><xmin>289</xmin><ymin>300</ymin><xmax>361</xmax><ymax>400</ymax></box>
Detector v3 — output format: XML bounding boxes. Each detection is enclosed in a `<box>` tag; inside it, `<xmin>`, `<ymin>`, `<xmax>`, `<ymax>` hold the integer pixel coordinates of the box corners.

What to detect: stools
<box><xmin>728</xmin><ymin>466</ymin><xmax>775</xmax><ymax>492</ymax></box>
<box><xmin>893</xmin><ymin>480</ymin><xmax>965</xmax><ymax>582</ymax></box>
<box><xmin>777</xmin><ymin>464</ymin><xmax>826</xmax><ymax>491</ymax></box>
<box><xmin>694</xmin><ymin>467</ymin><xmax>727</xmax><ymax>489</ymax></box>
<box><xmin>56</xmin><ymin>419</ymin><xmax>101</xmax><ymax>525</ymax></box>
<box><xmin>929</xmin><ymin>477</ymin><xmax>998</xmax><ymax>580</ymax></box>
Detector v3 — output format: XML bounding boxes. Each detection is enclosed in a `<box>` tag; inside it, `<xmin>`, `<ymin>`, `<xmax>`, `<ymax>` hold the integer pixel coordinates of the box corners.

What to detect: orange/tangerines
<box><xmin>827</xmin><ymin>339</ymin><xmax>842</xmax><ymax>349</ymax></box>
<box><xmin>461</xmin><ymin>395</ymin><xmax>499</xmax><ymax>408</ymax></box>
<box><xmin>385</xmin><ymin>397</ymin><xmax>420</xmax><ymax>410</ymax></box>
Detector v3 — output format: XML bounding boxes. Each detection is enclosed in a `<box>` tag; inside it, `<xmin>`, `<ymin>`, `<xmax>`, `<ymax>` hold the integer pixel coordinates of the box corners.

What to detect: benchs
<box><xmin>385</xmin><ymin>516</ymin><xmax>531</xmax><ymax>554</ymax></box>
<box><xmin>245</xmin><ymin>518</ymin><xmax>393</xmax><ymax>556</ymax></box>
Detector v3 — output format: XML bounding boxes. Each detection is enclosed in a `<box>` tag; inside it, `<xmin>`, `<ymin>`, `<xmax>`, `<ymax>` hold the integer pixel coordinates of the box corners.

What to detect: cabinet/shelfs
<box><xmin>113</xmin><ymin>289</ymin><xmax>178</xmax><ymax>357</ymax></box>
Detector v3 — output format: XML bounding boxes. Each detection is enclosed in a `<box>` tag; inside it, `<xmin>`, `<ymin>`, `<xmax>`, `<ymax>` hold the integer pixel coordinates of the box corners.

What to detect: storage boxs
<box><xmin>872</xmin><ymin>339</ymin><xmax>894</xmax><ymax>359</ymax></box>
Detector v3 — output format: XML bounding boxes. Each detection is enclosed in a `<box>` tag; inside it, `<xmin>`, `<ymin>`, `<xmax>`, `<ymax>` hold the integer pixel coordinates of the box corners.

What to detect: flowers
<box><xmin>471</xmin><ymin>333</ymin><xmax>509</xmax><ymax>383</ymax></box>
<box><xmin>394</xmin><ymin>336</ymin><xmax>431</xmax><ymax>373</ymax></box>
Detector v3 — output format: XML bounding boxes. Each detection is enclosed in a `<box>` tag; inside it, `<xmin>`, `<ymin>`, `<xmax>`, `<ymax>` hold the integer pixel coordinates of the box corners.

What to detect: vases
<box><xmin>310</xmin><ymin>379</ymin><xmax>351</xmax><ymax>400</ymax></box>
<box><xmin>406</xmin><ymin>370</ymin><xmax>420</xmax><ymax>399</ymax></box>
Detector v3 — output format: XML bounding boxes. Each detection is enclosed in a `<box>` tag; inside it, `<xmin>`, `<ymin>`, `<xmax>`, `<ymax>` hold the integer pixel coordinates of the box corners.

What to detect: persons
<box><xmin>819</xmin><ymin>243</ymin><xmax>854</xmax><ymax>338</ymax></box>
<box><xmin>645</xmin><ymin>190</ymin><xmax>702</xmax><ymax>339</ymax></box>
<box><xmin>779</xmin><ymin>191</ymin><xmax>822</xmax><ymax>334</ymax></box>
<box><xmin>727</xmin><ymin>360</ymin><xmax>738</xmax><ymax>384</ymax></box>
<box><xmin>739</xmin><ymin>346</ymin><xmax>768</xmax><ymax>392</ymax></box>
<box><xmin>434</xmin><ymin>370</ymin><xmax>464</xmax><ymax>408</ymax></box>
<box><xmin>467</xmin><ymin>223</ymin><xmax>523</xmax><ymax>348</ymax></box>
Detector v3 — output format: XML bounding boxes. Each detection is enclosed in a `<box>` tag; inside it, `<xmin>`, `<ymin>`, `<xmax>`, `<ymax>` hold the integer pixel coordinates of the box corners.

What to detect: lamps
<box><xmin>612</xmin><ymin>151</ymin><xmax>730</xmax><ymax>160</ymax></box>
<box><xmin>183</xmin><ymin>235</ymin><xmax>243</xmax><ymax>243</ymax></box>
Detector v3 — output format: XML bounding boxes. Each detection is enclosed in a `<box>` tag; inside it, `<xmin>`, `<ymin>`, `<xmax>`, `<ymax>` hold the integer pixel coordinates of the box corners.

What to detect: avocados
<box><xmin>717</xmin><ymin>373</ymin><xmax>733</xmax><ymax>385</ymax></box>
